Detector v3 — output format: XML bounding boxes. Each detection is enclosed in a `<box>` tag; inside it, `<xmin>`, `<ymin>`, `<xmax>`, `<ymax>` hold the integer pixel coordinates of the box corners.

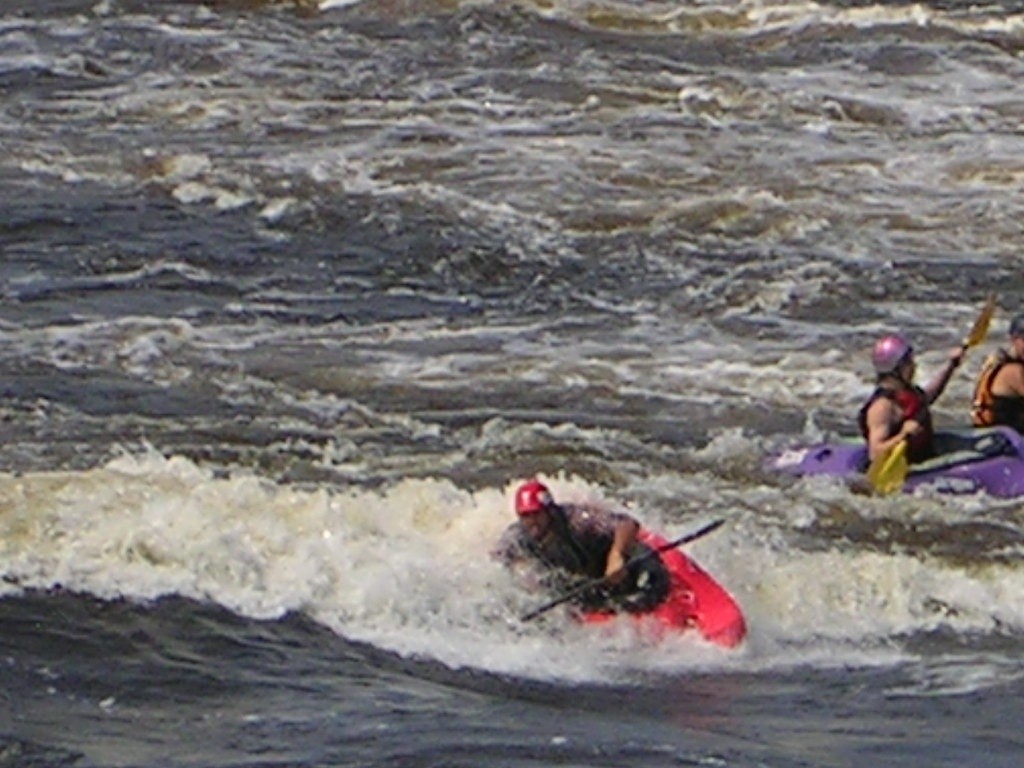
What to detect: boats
<box><xmin>769</xmin><ymin>425</ymin><xmax>1024</xmax><ymax>506</ymax></box>
<box><xmin>556</xmin><ymin>524</ymin><xmax>748</xmax><ymax>649</ymax></box>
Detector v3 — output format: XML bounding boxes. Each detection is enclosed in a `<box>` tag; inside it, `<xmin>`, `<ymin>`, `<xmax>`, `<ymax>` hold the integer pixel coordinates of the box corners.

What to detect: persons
<box><xmin>857</xmin><ymin>333</ymin><xmax>1024</xmax><ymax>475</ymax></box>
<box><xmin>969</xmin><ymin>314</ymin><xmax>1024</xmax><ymax>437</ymax></box>
<box><xmin>502</xmin><ymin>483</ymin><xmax>638</xmax><ymax>587</ymax></box>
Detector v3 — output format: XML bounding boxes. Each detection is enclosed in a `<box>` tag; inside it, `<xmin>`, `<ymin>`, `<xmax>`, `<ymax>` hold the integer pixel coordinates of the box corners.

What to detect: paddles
<box><xmin>859</xmin><ymin>285</ymin><xmax>1002</xmax><ymax>505</ymax></box>
<box><xmin>509</xmin><ymin>513</ymin><xmax>733</xmax><ymax>629</ymax></box>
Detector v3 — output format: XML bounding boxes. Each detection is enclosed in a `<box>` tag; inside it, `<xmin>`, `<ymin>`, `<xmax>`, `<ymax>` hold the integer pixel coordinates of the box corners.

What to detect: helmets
<box><xmin>1009</xmin><ymin>315</ymin><xmax>1024</xmax><ymax>341</ymax></box>
<box><xmin>517</xmin><ymin>480</ymin><xmax>553</xmax><ymax>514</ymax></box>
<box><xmin>872</xmin><ymin>335</ymin><xmax>914</xmax><ymax>376</ymax></box>
